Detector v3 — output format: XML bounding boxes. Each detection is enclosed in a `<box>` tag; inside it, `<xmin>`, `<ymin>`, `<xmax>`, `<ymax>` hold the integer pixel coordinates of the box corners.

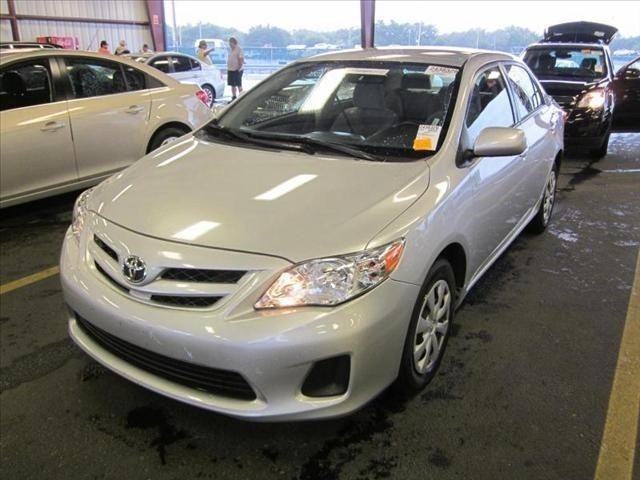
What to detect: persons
<box><xmin>138</xmin><ymin>44</ymin><xmax>152</xmax><ymax>53</ymax></box>
<box><xmin>226</xmin><ymin>38</ymin><xmax>244</xmax><ymax>103</ymax></box>
<box><xmin>197</xmin><ymin>40</ymin><xmax>214</xmax><ymax>65</ymax></box>
<box><xmin>114</xmin><ymin>40</ymin><xmax>130</xmax><ymax>55</ymax></box>
<box><xmin>98</xmin><ymin>40</ymin><xmax>112</xmax><ymax>55</ymax></box>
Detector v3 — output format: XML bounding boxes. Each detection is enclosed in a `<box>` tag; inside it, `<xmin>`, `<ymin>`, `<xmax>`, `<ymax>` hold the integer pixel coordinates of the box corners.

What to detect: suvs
<box><xmin>518</xmin><ymin>20</ymin><xmax>640</xmax><ymax>159</ymax></box>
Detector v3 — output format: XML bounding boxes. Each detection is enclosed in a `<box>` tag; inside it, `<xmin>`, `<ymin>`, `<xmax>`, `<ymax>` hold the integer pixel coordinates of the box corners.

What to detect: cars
<box><xmin>118</xmin><ymin>50</ymin><xmax>225</xmax><ymax>110</ymax></box>
<box><xmin>0</xmin><ymin>47</ymin><xmax>216</xmax><ymax>210</ymax></box>
<box><xmin>59</xmin><ymin>45</ymin><xmax>566</xmax><ymax>423</ymax></box>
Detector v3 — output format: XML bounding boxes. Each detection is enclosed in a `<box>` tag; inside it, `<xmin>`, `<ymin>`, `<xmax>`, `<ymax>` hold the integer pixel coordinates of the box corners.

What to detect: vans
<box><xmin>194</xmin><ymin>39</ymin><xmax>228</xmax><ymax>64</ymax></box>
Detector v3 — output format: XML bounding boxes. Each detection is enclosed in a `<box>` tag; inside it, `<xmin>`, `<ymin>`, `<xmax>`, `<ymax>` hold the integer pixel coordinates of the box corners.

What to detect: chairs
<box><xmin>572</xmin><ymin>56</ymin><xmax>601</xmax><ymax>78</ymax></box>
<box><xmin>1</xmin><ymin>65</ymin><xmax>26</xmax><ymax>110</ymax></box>
<box><xmin>330</xmin><ymin>73</ymin><xmax>502</xmax><ymax>138</ymax></box>
<box><xmin>67</xmin><ymin>63</ymin><xmax>141</xmax><ymax>98</ymax></box>
<box><xmin>538</xmin><ymin>54</ymin><xmax>557</xmax><ymax>75</ymax></box>
